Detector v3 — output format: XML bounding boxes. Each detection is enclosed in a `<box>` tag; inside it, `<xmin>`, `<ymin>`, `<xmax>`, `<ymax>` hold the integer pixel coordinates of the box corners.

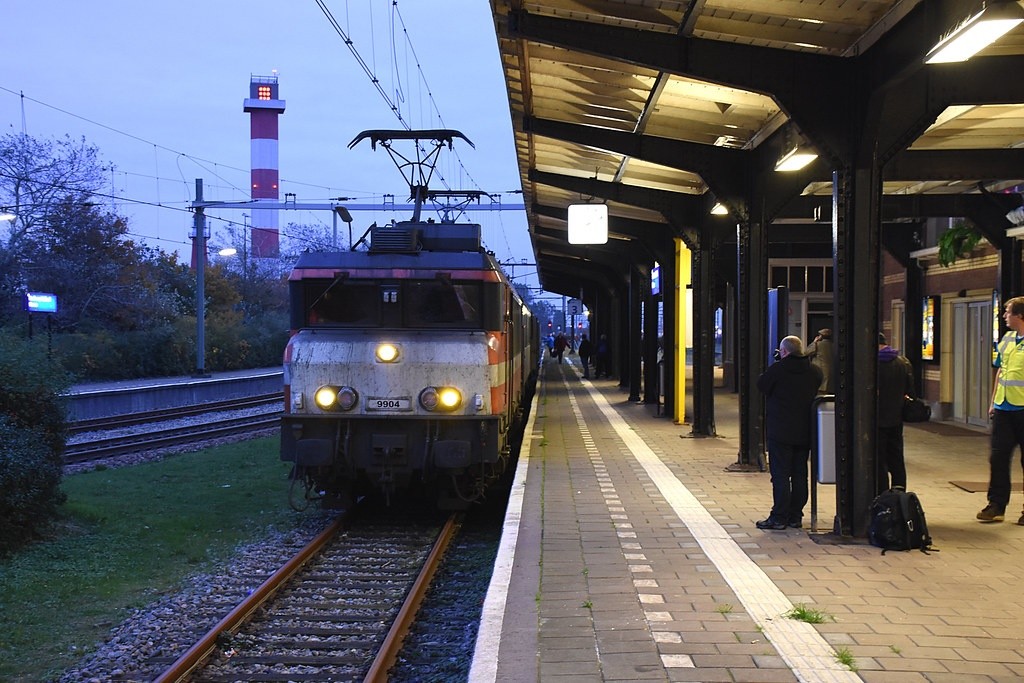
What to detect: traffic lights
<box><xmin>579</xmin><ymin>324</ymin><xmax>582</xmax><ymax>328</ymax></box>
<box><xmin>558</xmin><ymin>326</ymin><xmax>561</xmax><ymax>330</ymax></box>
<box><xmin>548</xmin><ymin>323</ymin><xmax>551</xmax><ymax>327</ymax></box>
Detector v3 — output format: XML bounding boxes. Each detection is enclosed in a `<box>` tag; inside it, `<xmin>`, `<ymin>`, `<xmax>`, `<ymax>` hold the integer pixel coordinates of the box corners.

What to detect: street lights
<box><xmin>334</xmin><ymin>205</ymin><xmax>354</xmax><ymax>249</ymax></box>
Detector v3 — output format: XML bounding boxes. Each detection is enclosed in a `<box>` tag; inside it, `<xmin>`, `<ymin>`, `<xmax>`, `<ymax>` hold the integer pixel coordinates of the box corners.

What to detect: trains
<box><xmin>280</xmin><ymin>218</ymin><xmax>542</xmax><ymax>509</ymax></box>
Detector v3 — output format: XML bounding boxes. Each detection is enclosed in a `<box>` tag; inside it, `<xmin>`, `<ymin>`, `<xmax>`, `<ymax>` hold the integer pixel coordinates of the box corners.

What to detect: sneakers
<box><xmin>1018</xmin><ymin>515</ymin><xmax>1024</xmax><ymax>524</ymax></box>
<box><xmin>977</xmin><ymin>504</ymin><xmax>1004</xmax><ymax>520</ymax></box>
<box><xmin>756</xmin><ymin>515</ymin><xmax>785</xmax><ymax>529</ymax></box>
<box><xmin>784</xmin><ymin>513</ymin><xmax>802</xmax><ymax>528</ymax></box>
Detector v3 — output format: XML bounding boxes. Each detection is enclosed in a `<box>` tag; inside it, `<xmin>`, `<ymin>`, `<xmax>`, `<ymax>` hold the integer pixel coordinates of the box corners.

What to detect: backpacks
<box><xmin>904</xmin><ymin>397</ymin><xmax>931</xmax><ymax>422</ymax></box>
<box><xmin>868</xmin><ymin>486</ymin><xmax>929</xmax><ymax>550</ymax></box>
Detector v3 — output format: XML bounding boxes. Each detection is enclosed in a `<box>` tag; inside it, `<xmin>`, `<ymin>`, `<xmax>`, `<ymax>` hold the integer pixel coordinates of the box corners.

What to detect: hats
<box><xmin>818</xmin><ymin>329</ymin><xmax>833</xmax><ymax>336</ymax></box>
<box><xmin>878</xmin><ymin>332</ymin><xmax>887</xmax><ymax>344</ymax></box>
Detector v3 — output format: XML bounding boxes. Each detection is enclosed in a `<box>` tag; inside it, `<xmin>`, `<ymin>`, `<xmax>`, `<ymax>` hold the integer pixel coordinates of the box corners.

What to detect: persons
<box><xmin>546</xmin><ymin>332</ymin><xmax>609</xmax><ymax>380</ymax></box>
<box><xmin>803</xmin><ymin>328</ymin><xmax>833</xmax><ymax>396</ymax></box>
<box><xmin>878</xmin><ymin>332</ymin><xmax>912</xmax><ymax>497</ymax></box>
<box><xmin>978</xmin><ymin>296</ymin><xmax>1024</xmax><ymax>526</ymax></box>
<box><xmin>757</xmin><ymin>335</ymin><xmax>823</xmax><ymax>530</ymax></box>
